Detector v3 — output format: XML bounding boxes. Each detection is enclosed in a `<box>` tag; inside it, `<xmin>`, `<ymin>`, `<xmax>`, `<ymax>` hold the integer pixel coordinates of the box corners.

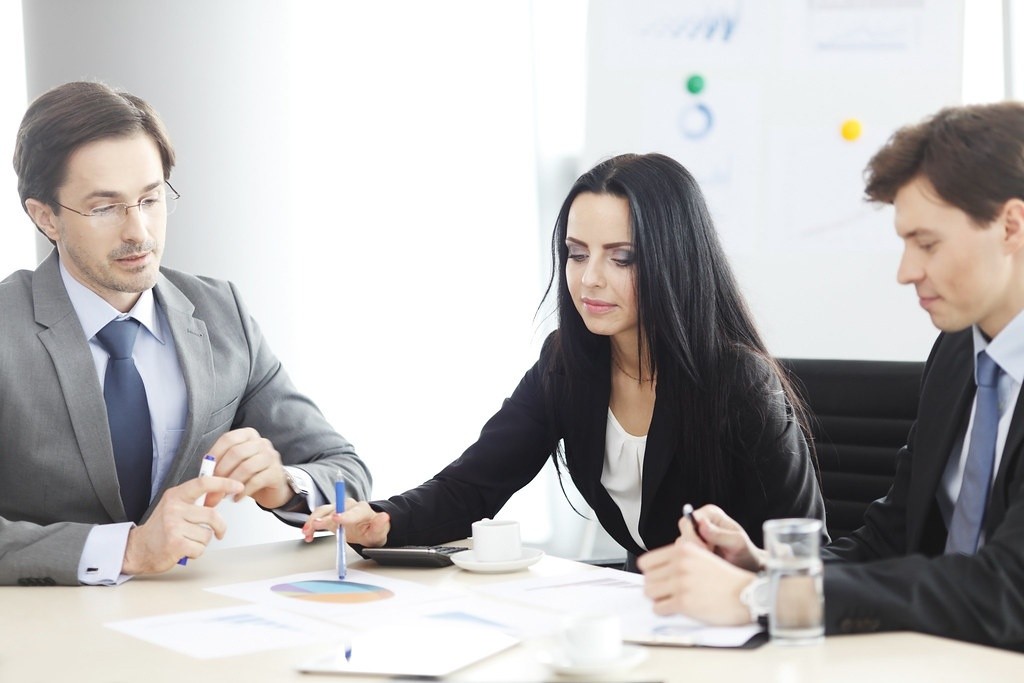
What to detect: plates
<box><xmin>449</xmin><ymin>548</ymin><xmax>545</xmax><ymax>573</ymax></box>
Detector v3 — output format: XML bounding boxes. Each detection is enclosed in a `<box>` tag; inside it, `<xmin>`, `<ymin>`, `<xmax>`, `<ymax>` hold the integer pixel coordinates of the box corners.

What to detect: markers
<box><xmin>178</xmin><ymin>454</ymin><xmax>218</xmax><ymax>567</ymax></box>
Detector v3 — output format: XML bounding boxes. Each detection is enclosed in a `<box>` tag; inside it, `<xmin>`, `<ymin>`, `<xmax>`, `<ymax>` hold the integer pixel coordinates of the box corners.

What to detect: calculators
<box><xmin>363</xmin><ymin>544</ymin><xmax>467</xmax><ymax>566</ymax></box>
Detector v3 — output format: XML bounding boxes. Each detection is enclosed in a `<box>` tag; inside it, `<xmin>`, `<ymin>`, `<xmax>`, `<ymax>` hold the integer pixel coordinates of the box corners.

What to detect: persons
<box><xmin>302</xmin><ymin>152</ymin><xmax>830</xmax><ymax>575</ymax></box>
<box><xmin>1</xmin><ymin>80</ymin><xmax>376</xmax><ymax>586</ymax></box>
<box><xmin>638</xmin><ymin>96</ymin><xmax>1023</xmax><ymax>654</ymax></box>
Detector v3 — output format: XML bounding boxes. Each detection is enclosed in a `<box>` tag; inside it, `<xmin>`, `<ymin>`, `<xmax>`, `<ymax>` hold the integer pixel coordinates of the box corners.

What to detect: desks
<box><xmin>0</xmin><ymin>533</ymin><xmax>1024</xmax><ymax>683</ymax></box>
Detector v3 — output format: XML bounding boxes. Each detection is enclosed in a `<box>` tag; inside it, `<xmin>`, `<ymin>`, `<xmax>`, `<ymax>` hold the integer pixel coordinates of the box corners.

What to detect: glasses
<box><xmin>51</xmin><ymin>180</ymin><xmax>180</xmax><ymax>230</ymax></box>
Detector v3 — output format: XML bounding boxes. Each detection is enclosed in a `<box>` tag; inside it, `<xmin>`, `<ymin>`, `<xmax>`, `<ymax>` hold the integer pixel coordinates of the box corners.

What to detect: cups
<box><xmin>472</xmin><ymin>519</ymin><xmax>522</xmax><ymax>562</ymax></box>
<box><xmin>763</xmin><ymin>518</ymin><xmax>827</xmax><ymax>640</ymax></box>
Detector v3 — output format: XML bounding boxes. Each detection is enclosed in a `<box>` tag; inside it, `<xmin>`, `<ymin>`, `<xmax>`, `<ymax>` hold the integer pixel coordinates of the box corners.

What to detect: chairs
<box><xmin>775</xmin><ymin>357</ymin><xmax>928</xmax><ymax>543</ymax></box>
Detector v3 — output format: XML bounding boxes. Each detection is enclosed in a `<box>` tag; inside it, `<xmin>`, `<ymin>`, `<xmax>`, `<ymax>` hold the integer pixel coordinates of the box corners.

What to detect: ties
<box><xmin>943</xmin><ymin>349</ymin><xmax>1007</xmax><ymax>559</ymax></box>
<box><xmin>95</xmin><ymin>318</ymin><xmax>153</xmax><ymax>527</ymax></box>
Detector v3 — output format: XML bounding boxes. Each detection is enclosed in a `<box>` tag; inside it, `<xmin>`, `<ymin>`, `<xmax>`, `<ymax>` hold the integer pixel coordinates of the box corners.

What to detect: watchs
<box><xmin>739</xmin><ymin>576</ymin><xmax>770</xmax><ymax>625</ymax></box>
<box><xmin>275</xmin><ymin>467</ymin><xmax>310</xmax><ymax>512</ymax></box>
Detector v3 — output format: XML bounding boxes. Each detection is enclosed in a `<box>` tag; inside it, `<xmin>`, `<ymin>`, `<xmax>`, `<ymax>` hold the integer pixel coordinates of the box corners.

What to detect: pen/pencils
<box><xmin>335</xmin><ymin>469</ymin><xmax>347</xmax><ymax>580</ymax></box>
<box><xmin>681</xmin><ymin>504</ymin><xmax>705</xmax><ymax>541</ymax></box>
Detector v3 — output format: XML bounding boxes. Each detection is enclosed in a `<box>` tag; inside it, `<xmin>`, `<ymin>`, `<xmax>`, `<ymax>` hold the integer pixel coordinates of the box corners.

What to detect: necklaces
<box><xmin>613</xmin><ymin>358</ymin><xmax>656</xmax><ymax>382</ymax></box>
